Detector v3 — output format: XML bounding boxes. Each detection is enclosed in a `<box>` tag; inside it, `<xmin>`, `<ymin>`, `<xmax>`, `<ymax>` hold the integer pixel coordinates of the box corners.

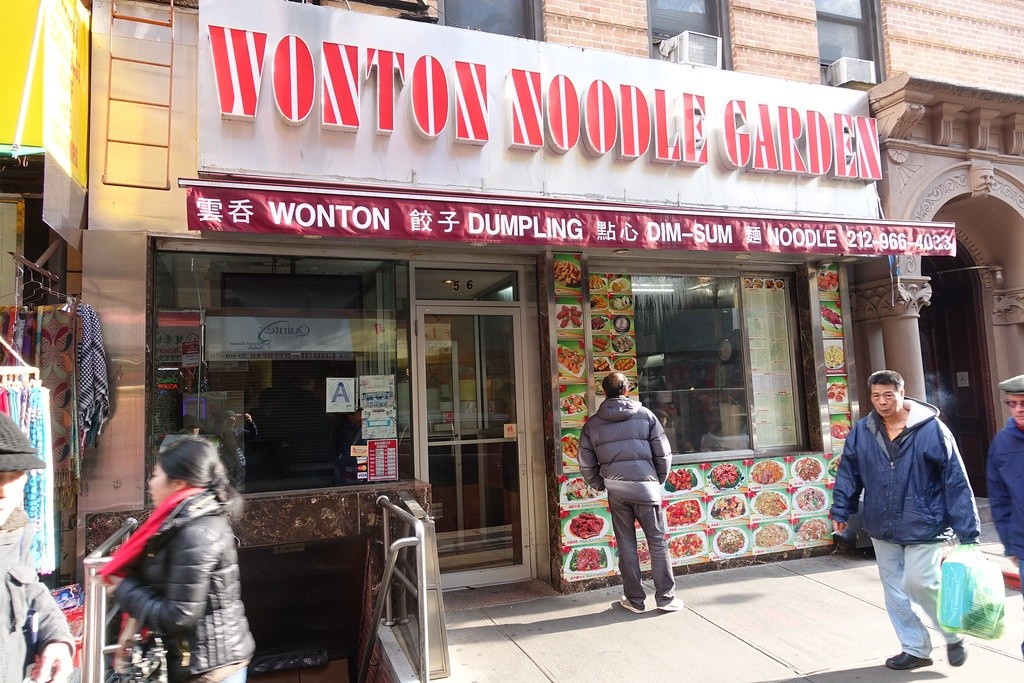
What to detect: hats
<box><xmin>997</xmin><ymin>374</ymin><xmax>1024</xmax><ymax>394</ymax></box>
<box><xmin>0</xmin><ymin>413</ymin><xmax>45</xmax><ymax>472</ymax></box>
<box><xmin>220</xmin><ymin>410</ymin><xmax>242</xmax><ymax>420</ymax></box>
<box><xmin>181</xmin><ymin>415</ymin><xmax>203</xmax><ymax>429</ymax></box>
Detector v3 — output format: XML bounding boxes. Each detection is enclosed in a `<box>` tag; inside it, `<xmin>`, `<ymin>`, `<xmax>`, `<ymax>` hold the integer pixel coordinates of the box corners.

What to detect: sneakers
<box><xmin>620</xmin><ymin>595</ymin><xmax>645</xmax><ymax>613</ymax></box>
<box><xmin>657</xmin><ymin>598</ymin><xmax>684</xmax><ymax>611</ymax></box>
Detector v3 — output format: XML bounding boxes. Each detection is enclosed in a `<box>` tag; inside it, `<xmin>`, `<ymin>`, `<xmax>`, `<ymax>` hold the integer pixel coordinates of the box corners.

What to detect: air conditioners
<box><xmin>665</xmin><ymin>31</ymin><xmax>722</xmax><ymax>68</ymax></box>
<box><xmin>827</xmin><ymin>57</ymin><xmax>875</xmax><ymax>87</ymax></box>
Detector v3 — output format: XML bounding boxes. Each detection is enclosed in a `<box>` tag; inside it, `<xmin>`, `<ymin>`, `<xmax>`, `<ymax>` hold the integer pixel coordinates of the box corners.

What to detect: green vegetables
<box><xmin>688</xmin><ymin>469</ymin><xmax>697</xmax><ymax>487</ymax></box>
<box><xmin>710</xmin><ymin>470</ymin><xmax>720</xmax><ymax>489</ymax></box>
<box><xmin>726</xmin><ymin>468</ymin><xmax>741</xmax><ymax>487</ymax></box>
<box><xmin>570</xmin><ymin>549</ymin><xmax>579</xmax><ymax>570</ymax></box>
<box><xmin>665</xmin><ymin>482</ymin><xmax>675</xmax><ymax>492</ymax></box>
<box><xmin>599</xmin><ymin>548</ymin><xmax>606</xmax><ymax>568</ymax></box>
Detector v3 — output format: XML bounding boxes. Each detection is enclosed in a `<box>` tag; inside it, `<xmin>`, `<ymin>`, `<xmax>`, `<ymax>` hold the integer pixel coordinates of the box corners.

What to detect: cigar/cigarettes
<box><xmin>831</xmin><ymin>531</ymin><xmax>839</xmax><ymax>535</ymax></box>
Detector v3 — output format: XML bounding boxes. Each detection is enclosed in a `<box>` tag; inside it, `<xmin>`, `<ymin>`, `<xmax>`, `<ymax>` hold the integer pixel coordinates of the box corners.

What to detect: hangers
<box><xmin>0</xmin><ymin>254</ymin><xmax>82</xmax><ymax>306</ymax></box>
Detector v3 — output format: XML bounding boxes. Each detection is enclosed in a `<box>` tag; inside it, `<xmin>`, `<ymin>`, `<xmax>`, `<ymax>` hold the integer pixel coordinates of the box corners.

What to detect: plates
<box><xmin>668</xmin><ymin>531</ymin><xmax>708</xmax><ymax>561</ymax></box>
<box><xmin>750</xmin><ymin>491</ymin><xmax>790</xmax><ymax>517</ymax></box>
<box><xmin>706</xmin><ymin>464</ymin><xmax>745</xmax><ymax>491</ymax></box>
<box><xmin>664</xmin><ymin>498</ymin><xmax>706</xmax><ymax>528</ymax></box>
<box><xmin>564</xmin><ymin>547</ymin><xmax>614</xmax><ymax>572</ymax></box>
<box><xmin>790</xmin><ymin>457</ymin><xmax>826</xmax><ymax>483</ymax></box>
<box><xmin>553</xmin><ymin>252</ymin><xmax>638</xmax><ymax>463</ymax></box>
<box><xmin>713</xmin><ymin>527</ymin><xmax>748</xmax><ymax>558</ymax></box>
<box><xmin>817</xmin><ymin>270</ymin><xmax>854</xmax><ymax>446</ymax></box>
<box><xmin>745</xmin><ymin>278</ymin><xmax>784</xmax><ymax>289</ymax></box>
<box><xmin>564</xmin><ymin>515</ymin><xmax>609</xmax><ymax>541</ymax></box>
<box><xmin>709</xmin><ymin>495</ymin><xmax>748</xmax><ymax>520</ymax></box>
<box><xmin>794</xmin><ymin>517</ymin><xmax>832</xmax><ymax>543</ymax></box>
<box><xmin>828</xmin><ymin>453</ymin><xmax>842</xmax><ymax>477</ymax></box>
<box><xmin>749</xmin><ymin>461</ymin><xmax>787</xmax><ymax>487</ymax></box>
<box><xmin>752</xmin><ymin>522</ymin><xmax>792</xmax><ymax>550</ymax></box>
<box><xmin>661</xmin><ymin>468</ymin><xmax>704</xmax><ymax>497</ymax></box>
<box><xmin>560</xmin><ymin>476</ymin><xmax>607</xmax><ymax>505</ymax></box>
<box><xmin>792</xmin><ymin>486</ymin><xmax>828</xmax><ymax>513</ymax></box>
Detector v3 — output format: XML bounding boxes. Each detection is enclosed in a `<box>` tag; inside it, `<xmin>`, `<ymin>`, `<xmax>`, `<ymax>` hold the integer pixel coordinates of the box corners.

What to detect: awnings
<box><xmin>0</xmin><ymin>0</ymin><xmax>91</xmax><ymax>194</ymax></box>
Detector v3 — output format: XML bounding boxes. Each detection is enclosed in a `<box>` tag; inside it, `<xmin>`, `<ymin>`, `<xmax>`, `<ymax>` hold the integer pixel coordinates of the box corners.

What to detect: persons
<box><xmin>95</xmin><ymin>438</ymin><xmax>257</xmax><ymax>683</ymax></box>
<box><xmin>655</xmin><ymin>409</ymin><xmax>667</xmax><ymax>428</ymax></box>
<box><xmin>828</xmin><ymin>370</ymin><xmax>982</xmax><ymax>670</ymax></box>
<box><xmin>653</xmin><ymin>390</ymin><xmax>679</xmax><ymax>454</ymax></box>
<box><xmin>333</xmin><ymin>409</ymin><xmax>367</xmax><ymax>486</ymax></box>
<box><xmin>684</xmin><ymin>365</ymin><xmax>706</xmax><ymax>455</ymax></box>
<box><xmin>185</xmin><ymin>409</ymin><xmax>258</xmax><ymax>489</ymax></box>
<box><xmin>577</xmin><ymin>372</ymin><xmax>685</xmax><ymax>613</ymax></box>
<box><xmin>0</xmin><ymin>410</ymin><xmax>74</xmax><ymax>683</ymax></box>
<box><xmin>988</xmin><ymin>374</ymin><xmax>1024</xmax><ymax>664</ymax></box>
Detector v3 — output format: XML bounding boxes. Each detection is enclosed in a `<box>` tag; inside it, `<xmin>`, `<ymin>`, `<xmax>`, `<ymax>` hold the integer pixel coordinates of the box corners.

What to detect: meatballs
<box><xmin>617</xmin><ymin>359</ymin><xmax>633</xmax><ymax>370</ymax></box>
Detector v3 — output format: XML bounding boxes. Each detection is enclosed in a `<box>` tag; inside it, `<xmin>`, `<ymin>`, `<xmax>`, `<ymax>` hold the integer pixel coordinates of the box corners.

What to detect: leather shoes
<box><xmin>886</xmin><ymin>652</ymin><xmax>933</xmax><ymax>669</ymax></box>
<box><xmin>947</xmin><ymin>639</ymin><xmax>967</xmax><ymax>667</ymax></box>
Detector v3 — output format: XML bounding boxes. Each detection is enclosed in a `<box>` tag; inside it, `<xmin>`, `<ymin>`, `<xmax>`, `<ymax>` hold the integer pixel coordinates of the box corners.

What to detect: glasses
<box><xmin>1003</xmin><ymin>401</ymin><xmax>1024</xmax><ymax>408</ymax></box>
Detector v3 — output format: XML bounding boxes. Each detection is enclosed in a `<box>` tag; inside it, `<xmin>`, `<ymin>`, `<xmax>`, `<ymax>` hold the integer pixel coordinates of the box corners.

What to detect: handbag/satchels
<box><xmin>104</xmin><ymin>599</ymin><xmax>162</xmax><ymax>683</ymax></box>
<box><xmin>937</xmin><ymin>544</ymin><xmax>1005</xmax><ymax>640</ymax></box>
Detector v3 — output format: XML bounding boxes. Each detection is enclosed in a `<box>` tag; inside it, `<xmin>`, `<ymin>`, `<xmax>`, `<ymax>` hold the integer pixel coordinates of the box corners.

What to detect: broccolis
<box><xmin>568</xmin><ymin>492</ymin><xmax>579</xmax><ymax>500</ymax></box>
